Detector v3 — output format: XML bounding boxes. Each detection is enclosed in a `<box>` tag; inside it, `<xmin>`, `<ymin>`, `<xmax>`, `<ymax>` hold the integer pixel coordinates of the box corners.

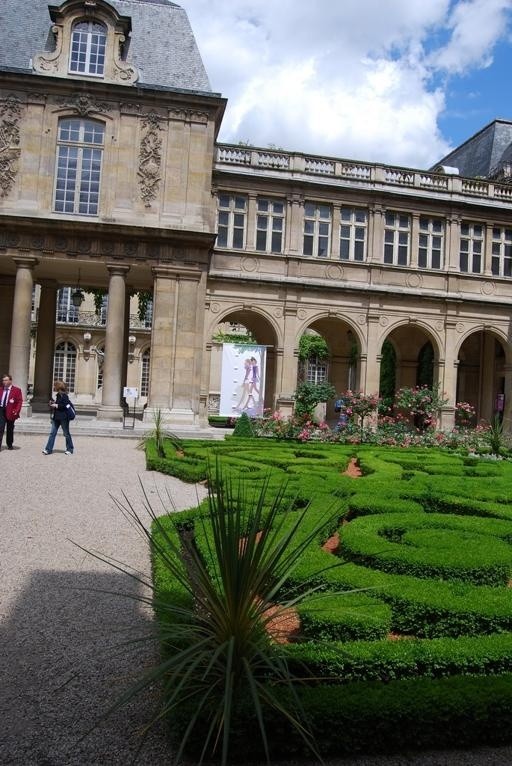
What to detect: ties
<box><xmin>2</xmin><ymin>389</ymin><xmax>8</xmax><ymax>409</ymax></box>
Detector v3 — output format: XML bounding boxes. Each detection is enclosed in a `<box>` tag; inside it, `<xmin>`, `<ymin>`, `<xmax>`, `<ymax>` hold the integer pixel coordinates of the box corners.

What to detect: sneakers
<box><xmin>64</xmin><ymin>451</ymin><xmax>73</xmax><ymax>455</ymax></box>
<box><xmin>41</xmin><ymin>449</ymin><xmax>53</xmax><ymax>455</ymax></box>
<box><xmin>8</xmin><ymin>444</ymin><xmax>13</xmax><ymax>450</ymax></box>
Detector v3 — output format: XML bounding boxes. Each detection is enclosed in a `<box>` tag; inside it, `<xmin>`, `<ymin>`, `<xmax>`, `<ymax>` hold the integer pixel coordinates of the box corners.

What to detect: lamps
<box><xmin>71</xmin><ymin>279</ymin><xmax>84</xmax><ymax>306</ymax></box>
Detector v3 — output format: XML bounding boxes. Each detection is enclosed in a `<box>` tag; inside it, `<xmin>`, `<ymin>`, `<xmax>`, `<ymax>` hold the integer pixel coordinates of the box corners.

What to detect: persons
<box><xmin>242</xmin><ymin>357</ymin><xmax>260</xmax><ymax>410</ymax></box>
<box><xmin>0</xmin><ymin>374</ymin><xmax>22</xmax><ymax>452</ymax></box>
<box><xmin>232</xmin><ymin>359</ymin><xmax>255</xmax><ymax>409</ymax></box>
<box><xmin>42</xmin><ymin>381</ymin><xmax>75</xmax><ymax>455</ymax></box>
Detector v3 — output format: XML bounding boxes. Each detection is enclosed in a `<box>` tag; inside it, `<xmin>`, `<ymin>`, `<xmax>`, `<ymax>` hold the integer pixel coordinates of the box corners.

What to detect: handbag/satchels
<box><xmin>66</xmin><ymin>400</ymin><xmax>76</xmax><ymax>420</ymax></box>
